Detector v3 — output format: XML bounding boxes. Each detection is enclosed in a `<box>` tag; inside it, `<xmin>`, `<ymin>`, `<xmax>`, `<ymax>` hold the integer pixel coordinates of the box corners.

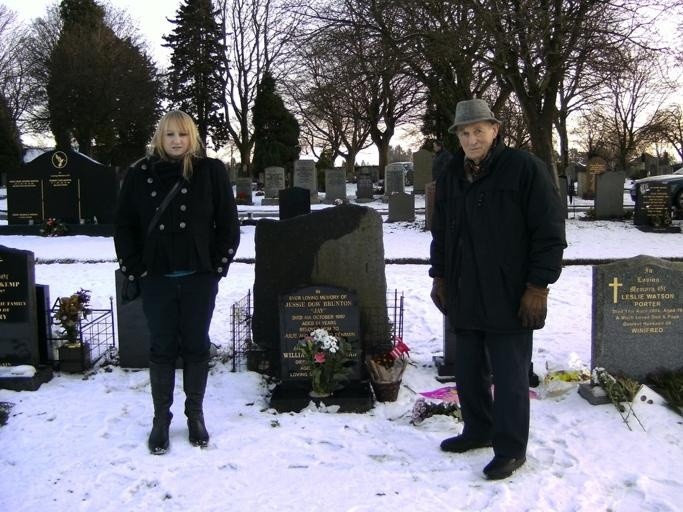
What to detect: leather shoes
<box><xmin>438</xmin><ymin>432</ymin><xmax>492</xmax><ymax>454</ymax></box>
<box><xmin>482</xmin><ymin>451</ymin><xmax>527</xmax><ymax>479</ymax></box>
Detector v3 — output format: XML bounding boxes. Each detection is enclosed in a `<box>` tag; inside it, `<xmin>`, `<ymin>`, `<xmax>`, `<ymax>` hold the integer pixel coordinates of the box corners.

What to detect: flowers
<box><xmin>407</xmin><ymin>395</ymin><xmax>461</xmax><ymax>427</ymax></box>
<box><xmin>292</xmin><ymin>322</ymin><xmax>348</xmax><ymax>393</ymax></box>
<box><xmin>585</xmin><ymin>361</ymin><xmax>647</xmax><ymax>432</ymax></box>
<box><xmin>41</xmin><ymin>217</ymin><xmax>67</xmax><ymax>237</ymax></box>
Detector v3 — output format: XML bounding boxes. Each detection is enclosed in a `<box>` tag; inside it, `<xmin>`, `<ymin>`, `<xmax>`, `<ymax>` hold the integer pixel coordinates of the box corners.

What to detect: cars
<box><xmin>628</xmin><ymin>167</ymin><xmax>683</xmax><ymax>218</ymax></box>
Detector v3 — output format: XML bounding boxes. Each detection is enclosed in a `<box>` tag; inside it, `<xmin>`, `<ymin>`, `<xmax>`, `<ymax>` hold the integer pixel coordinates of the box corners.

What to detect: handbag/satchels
<box><xmin>119</xmin><ymin>277</ymin><xmax>141</xmax><ymax>306</ymax></box>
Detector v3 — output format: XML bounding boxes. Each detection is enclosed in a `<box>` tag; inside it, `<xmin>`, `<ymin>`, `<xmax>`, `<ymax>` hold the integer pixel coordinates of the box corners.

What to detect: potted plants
<box><xmin>51</xmin><ymin>287</ymin><xmax>93</xmax><ymax>380</ymax></box>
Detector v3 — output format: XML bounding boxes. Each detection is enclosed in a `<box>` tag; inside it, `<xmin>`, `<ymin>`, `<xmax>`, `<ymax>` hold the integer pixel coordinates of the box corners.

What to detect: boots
<box><xmin>182</xmin><ymin>357</ymin><xmax>211</xmax><ymax>449</ymax></box>
<box><xmin>144</xmin><ymin>356</ymin><xmax>179</xmax><ymax>456</ymax></box>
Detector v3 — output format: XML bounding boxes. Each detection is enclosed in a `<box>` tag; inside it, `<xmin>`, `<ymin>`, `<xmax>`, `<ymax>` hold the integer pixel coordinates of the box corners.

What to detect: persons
<box><xmin>427</xmin><ymin>97</ymin><xmax>566</xmax><ymax>481</ymax></box>
<box><xmin>111</xmin><ymin>108</ymin><xmax>241</xmax><ymax>453</ymax></box>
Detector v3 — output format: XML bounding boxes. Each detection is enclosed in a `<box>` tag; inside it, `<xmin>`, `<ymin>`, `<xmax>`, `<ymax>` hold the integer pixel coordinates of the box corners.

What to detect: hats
<box><xmin>448</xmin><ymin>98</ymin><xmax>500</xmax><ymax>132</ymax></box>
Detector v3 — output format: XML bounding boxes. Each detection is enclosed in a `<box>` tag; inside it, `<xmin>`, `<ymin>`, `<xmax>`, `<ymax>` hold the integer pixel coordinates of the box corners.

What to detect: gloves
<box><xmin>515</xmin><ymin>283</ymin><xmax>551</xmax><ymax>331</ymax></box>
<box><xmin>429</xmin><ymin>276</ymin><xmax>452</xmax><ymax>315</ymax></box>
